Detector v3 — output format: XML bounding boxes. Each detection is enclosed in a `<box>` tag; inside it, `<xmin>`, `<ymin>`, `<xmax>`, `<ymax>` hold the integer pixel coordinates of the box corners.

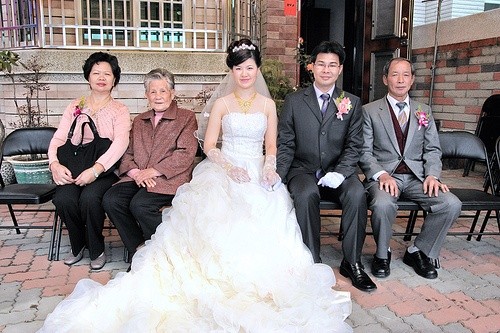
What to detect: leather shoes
<box><xmin>340</xmin><ymin>257</ymin><xmax>376</xmax><ymax>291</ymax></box>
<box><xmin>373</xmin><ymin>250</ymin><xmax>391</xmax><ymax>277</ymax></box>
<box><xmin>403</xmin><ymin>247</ymin><xmax>438</xmax><ymax>279</ymax></box>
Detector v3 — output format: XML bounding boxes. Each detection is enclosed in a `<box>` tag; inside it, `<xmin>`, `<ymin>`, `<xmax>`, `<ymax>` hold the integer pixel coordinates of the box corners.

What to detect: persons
<box><xmin>273</xmin><ymin>40</ymin><xmax>377</xmax><ymax>292</ymax></box>
<box><xmin>103</xmin><ymin>68</ymin><xmax>199</xmax><ymax>271</ymax></box>
<box><xmin>40</xmin><ymin>40</ymin><xmax>353</xmax><ymax>333</ymax></box>
<box><xmin>48</xmin><ymin>52</ymin><xmax>132</xmax><ymax>270</ymax></box>
<box><xmin>358</xmin><ymin>57</ymin><xmax>462</xmax><ymax>279</ymax></box>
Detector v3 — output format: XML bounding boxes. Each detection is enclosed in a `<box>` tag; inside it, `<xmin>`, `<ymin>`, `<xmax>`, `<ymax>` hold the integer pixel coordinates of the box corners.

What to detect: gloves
<box><xmin>317</xmin><ymin>172</ymin><xmax>345</xmax><ymax>189</ymax></box>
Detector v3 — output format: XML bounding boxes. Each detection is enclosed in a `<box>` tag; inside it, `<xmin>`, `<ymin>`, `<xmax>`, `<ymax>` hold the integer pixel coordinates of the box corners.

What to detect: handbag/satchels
<box><xmin>57</xmin><ymin>113</ymin><xmax>120</xmax><ymax>179</ymax></box>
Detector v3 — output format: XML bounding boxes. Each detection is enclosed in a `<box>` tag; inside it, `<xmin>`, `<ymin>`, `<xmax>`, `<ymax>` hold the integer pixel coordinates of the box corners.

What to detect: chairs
<box><xmin>320</xmin><ymin>94</ymin><xmax>500</xmax><ymax>269</ymax></box>
<box><xmin>0</xmin><ymin>127</ymin><xmax>60</xmax><ymax>261</ymax></box>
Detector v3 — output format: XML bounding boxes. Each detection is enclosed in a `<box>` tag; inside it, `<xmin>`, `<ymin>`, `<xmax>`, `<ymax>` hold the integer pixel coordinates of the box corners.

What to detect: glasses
<box><xmin>314</xmin><ymin>63</ymin><xmax>340</xmax><ymax>70</ymax></box>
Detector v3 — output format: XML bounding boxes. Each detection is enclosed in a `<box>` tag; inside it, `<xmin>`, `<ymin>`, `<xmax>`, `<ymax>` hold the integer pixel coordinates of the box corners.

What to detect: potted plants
<box><xmin>0</xmin><ymin>50</ymin><xmax>53</xmax><ymax>184</ymax></box>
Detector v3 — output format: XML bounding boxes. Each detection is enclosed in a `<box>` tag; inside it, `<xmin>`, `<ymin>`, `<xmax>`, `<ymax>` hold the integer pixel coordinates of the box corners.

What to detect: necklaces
<box><xmin>235</xmin><ymin>91</ymin><xmax>256</xmax><ymax>113</ymax></box>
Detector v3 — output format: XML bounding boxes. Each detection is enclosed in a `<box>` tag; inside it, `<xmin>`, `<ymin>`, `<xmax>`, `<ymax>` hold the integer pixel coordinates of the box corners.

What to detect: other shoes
<box><xmin>64</xmin><ymin>246</ymin><xmax>85</xmax><ymax>265</ymax></box>
<box><xmin>91</xmin><ymin>252</ymin><xmax>106</xmax><ymax>270</ymax></box>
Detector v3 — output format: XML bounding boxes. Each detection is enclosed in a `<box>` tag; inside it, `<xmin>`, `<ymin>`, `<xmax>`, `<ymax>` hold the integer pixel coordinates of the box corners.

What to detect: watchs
<box><xmin>91</xmin><ymin>167</ymin><xmax>99</xmax><ymax>177</ymax></box>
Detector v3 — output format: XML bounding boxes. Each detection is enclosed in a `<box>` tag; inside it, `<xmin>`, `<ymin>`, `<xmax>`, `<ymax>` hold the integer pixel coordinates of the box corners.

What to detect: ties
<box><xmin>396</xmin><ymin>102</ymin><xmax>408</xmax><ymax>133</ymax></box>
<box><xmin>316</xmin><ymin>94</ymin><xmax>329</xmax><ymax>179</ymax></box>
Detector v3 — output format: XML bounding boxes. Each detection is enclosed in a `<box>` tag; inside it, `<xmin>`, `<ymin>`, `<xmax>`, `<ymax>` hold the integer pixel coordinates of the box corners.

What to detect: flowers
<box><xmin>73</xmin><ymin>96</ymin><xmax>85</xmax><ymax>117</ymax></box>
<box><xmin>415</xmin><ymin>105</ymin><xmax>431</xmax><ymax>130</ymax></box>
<box><xmin>333</xmin><ymin>91</ymin><xmax>352</xmax><ymax>121</ymax></box>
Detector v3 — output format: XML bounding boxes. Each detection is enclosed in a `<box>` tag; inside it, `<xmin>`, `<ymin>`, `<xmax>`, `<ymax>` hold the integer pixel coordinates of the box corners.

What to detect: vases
<box><xmin>0</xmin><ymin>160</ymin><xmax>16</xmax><ymax>185</ymax></box>
